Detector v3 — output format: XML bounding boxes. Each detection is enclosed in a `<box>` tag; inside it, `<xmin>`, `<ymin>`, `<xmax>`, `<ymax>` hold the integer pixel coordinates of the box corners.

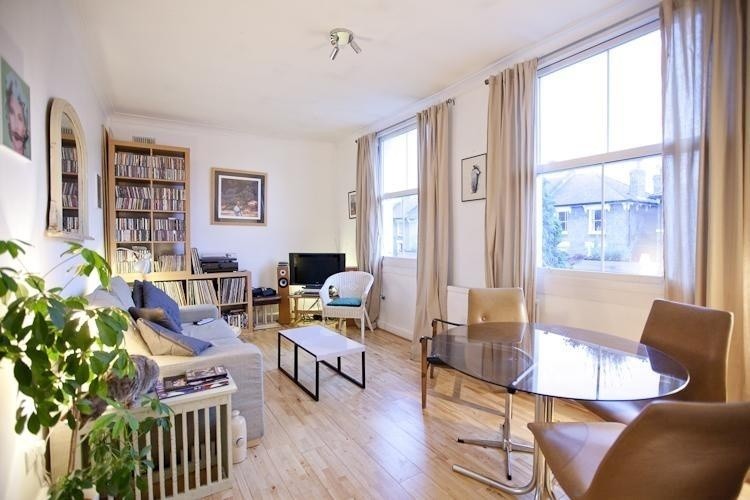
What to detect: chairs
<box><xmin>576</xmin><ymin>298</ymin><xmax>735</xmax><ymax>425</ymax></box>
<box><xmin>528</xmin><ymin>399</ymin><xmax>750</xmax><ymax>500</ymax></box>
<box><xmin>319</xmin><ymin>271</ymin><xmax>374</xmax><ymax>345</ymax></box>
<box><xmin>458</xmin><ymin>288</ymin><xmax>535</xmax><ymax>480</ymax></box>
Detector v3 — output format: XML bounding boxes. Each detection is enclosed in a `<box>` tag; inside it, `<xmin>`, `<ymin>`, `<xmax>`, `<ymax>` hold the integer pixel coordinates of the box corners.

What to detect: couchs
<box><xmin>85</xmin><ymin>276</ymin><xmax>266</xmax><ymax>465</ymax></box>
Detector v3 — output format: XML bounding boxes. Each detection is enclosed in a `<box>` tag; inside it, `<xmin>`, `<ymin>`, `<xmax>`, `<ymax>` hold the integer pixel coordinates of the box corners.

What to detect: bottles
<box><xmin>231</xmin><ymin>410</ymin><xmax>248</xmax><ymax>464</ymax></box>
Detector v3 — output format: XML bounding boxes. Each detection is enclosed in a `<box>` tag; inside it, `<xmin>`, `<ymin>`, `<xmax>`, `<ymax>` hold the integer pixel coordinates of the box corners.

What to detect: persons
<box><xmin>5</xmin><ymin>72</ymin><xmax>29</xmax><ymax>155</ymax></box>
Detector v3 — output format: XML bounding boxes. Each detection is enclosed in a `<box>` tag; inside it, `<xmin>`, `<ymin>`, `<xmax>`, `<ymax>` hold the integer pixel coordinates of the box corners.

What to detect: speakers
<box><xmin>277</xmin><ymin>266</ymin><xmax>291</xmax><ymax>324</ymax></box>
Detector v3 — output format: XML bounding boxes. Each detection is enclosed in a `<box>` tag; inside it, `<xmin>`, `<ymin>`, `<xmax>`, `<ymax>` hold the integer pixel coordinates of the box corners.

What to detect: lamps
<box><xmin>330</xmin><ymin>28</ymin><xmax>361</xmax><ymax>61</ymax></box>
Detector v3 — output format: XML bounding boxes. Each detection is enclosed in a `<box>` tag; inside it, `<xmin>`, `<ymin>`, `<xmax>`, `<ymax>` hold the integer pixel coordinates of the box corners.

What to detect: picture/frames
<box><xmin>348</xmin><ymin>191</ymin><xmax>357</xmax><ymax>218</ymax></box>
<box><xmin>461</xmin><ymin>153</ymin><xmax>487</xmax><ymax>202</ymax></box>
<box><xmin>210</xmin><ymin>167</ymin><xmax>268</xmax><ymax>227</ymax></box>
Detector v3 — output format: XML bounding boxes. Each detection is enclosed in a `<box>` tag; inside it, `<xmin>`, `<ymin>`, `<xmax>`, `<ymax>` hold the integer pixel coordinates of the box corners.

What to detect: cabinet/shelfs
<box><xmin>106</xmin><ymin>138</ymin><xmax>253</xmax><ymax>335</ymax></box>
<box><xmin>62</xmin><ymin>134</ymin><xmax>79</xmax><ymax>230</ymax></box>
<box><xmin>289</xmin><ymin>294</ymin><xmax>339</xmax><ymax>328</ymax></box>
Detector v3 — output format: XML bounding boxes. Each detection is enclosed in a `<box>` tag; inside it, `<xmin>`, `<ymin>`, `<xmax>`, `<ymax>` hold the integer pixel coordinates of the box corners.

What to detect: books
<box><xmin>61</xmin><ymin>145</ymin><xmax>78</xmax><ymax>233</ymax></box>
<box><xmin>114</xmin><ymin>151</ymin><xmax>249</xmax><ymax>330</ymax></box>
<box><xmin>153</xmin><ymin>364</ymin><xmax>231</xmax><ymax>400</ymax></box>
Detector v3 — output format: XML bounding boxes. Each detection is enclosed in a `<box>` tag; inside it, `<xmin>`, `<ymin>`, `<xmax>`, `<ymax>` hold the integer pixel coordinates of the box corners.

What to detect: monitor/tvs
<box><xmin>288</xmin><ymin>252</ymin><xmax>346</xmax><ymax>290</ymax></box>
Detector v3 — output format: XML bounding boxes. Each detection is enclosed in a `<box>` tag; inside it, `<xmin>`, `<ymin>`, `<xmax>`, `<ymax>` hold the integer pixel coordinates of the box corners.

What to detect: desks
<box><xmin>49</xmin><ymin>369</ymin><xmax>238</xmax><ymax>500</ymax></box>
<box><xmin>430</xmin><ymin>322</ymin><xmax>690</xmax><ymax>500</ymax></box>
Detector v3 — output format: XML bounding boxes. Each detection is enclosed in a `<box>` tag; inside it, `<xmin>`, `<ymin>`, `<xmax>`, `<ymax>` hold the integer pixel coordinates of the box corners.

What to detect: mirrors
<box><xmin>47</xmin><ymin>97</ymin><xmax>89</xmax><ymax>241</ymax></box>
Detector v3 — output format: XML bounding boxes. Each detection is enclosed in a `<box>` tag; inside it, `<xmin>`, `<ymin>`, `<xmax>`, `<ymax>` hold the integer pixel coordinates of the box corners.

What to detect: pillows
<box><xmin>128</xmin><ymin>280</ymin><xmax>212</xmax><ymax>356</ymax></box>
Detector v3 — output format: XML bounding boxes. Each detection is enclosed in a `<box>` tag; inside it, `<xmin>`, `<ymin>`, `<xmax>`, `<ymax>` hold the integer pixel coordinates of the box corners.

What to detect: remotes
<box><xmin>197</xmin><ymin>318</ymin><xmax>216</xmax><ymax>326</ymax></box>
